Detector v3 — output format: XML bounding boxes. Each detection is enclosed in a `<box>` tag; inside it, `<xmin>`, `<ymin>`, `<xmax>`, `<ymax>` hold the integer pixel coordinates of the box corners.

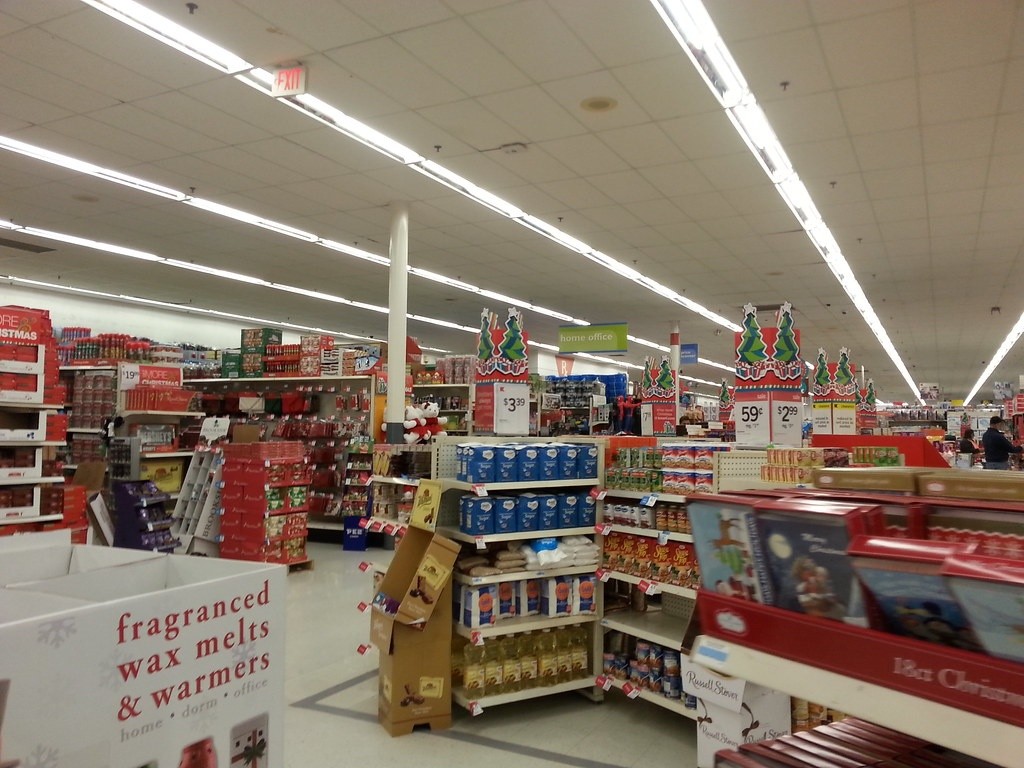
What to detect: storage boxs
<box><xmin>0</xmin><ymin>305</ymin><xmax>1024</xmax><ymax>768</ymax></box>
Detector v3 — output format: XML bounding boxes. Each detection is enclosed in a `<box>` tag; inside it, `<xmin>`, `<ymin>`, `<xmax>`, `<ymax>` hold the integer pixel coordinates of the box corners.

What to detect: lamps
<box><xmin>0</xmin><ymin>0</ymin><xmax>1024</xmax><ymax>404</ymax></box>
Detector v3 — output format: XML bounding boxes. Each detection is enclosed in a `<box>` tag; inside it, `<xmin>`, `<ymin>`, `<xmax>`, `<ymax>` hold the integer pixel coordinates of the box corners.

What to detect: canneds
<box><xmin>603</xmin><ymin>640</ymin><xmax>697</xmax><ymax>710</ymax></box>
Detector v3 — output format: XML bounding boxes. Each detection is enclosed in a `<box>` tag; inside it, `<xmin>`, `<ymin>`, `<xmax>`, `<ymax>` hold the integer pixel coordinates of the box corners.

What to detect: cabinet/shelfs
<box><xmin>62</xmin><ymin>363</ymin><xmax>207</xmax><ymax>499</ymax></box>
<box><xmin>185</xmin><ymin>374</ymin><xmax>411</xmax><ymax>534</ymax></box>
<box><xmin>172</xmin><ymin>450</ymin><xmax>220</xmax><ymax>544</ymax></box>
<box><xmin>1002</xmin><ymin>417</ymin><xmax>1013</xmax><ymax>438</ymax></box>
<box><xmin>889</xmin><ymin>420</ymin><xmax>948</xmax><ymax>434</ymax></box>
<box><xmin>600</xmin><ymin>450</ymin><xmax>769</xmax><ymax>721</ymax></box>
<box><xmin>0</xmin><ymin>403</ymin><xmax>68</xmax><ymax>525</ymax></box>
<box><xmin>359</xmin><ymin>434</ymin><xmax>605</xmax><ymax>712</ymax></box>
<box><xmin>411</xmin><ymin>383</ymin><xmax>538</xmax><ymax>438</ymax></box>
<box><xmin>560</xmin><ymin>395</ymin><xmax>610</xmax><ymax>435</ymax></box>
